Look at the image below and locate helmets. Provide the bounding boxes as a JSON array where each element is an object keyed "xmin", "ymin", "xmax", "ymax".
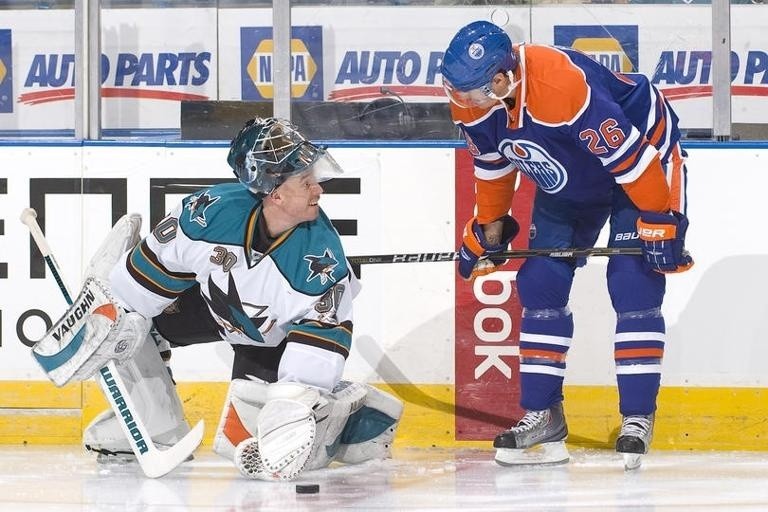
[
  {"xmin": 441, "ymin": 21, "xmax": 518, "ymax": 108},
  {"xmin": 227, "ymin": 117, "xmax": 344, "ymax": 195}
]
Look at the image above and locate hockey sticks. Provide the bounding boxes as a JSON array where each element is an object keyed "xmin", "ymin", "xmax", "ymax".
[{"xmin": 20, "ymin": 207, "xmax": 204, "ymax": 478}]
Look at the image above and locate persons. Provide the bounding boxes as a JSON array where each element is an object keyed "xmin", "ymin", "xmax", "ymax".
[
  {"xmin": 434, "ymin": 17, "xmax": 697, "ymax": 459},
  {"xmin": 79, "ymin": 114, "xmax": 401, "ymax": 483}
]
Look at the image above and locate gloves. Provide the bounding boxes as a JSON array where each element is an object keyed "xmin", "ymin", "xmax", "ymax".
[
  {"xmin": 636, "ymin": 211, "xmax": 694, "ymax": 273},
  {"xmin": 459, "ymin": 215, "xmax": 519, "ymax": 280}
]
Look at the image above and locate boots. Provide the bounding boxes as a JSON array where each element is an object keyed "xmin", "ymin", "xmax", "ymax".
[
  {"xmin": 616, "ymin": 411, "xmax": 655, "ymax": 455},
  {"xmin": 493, "ymin": 400, "xmax": 569, "ymax": 451}
]
